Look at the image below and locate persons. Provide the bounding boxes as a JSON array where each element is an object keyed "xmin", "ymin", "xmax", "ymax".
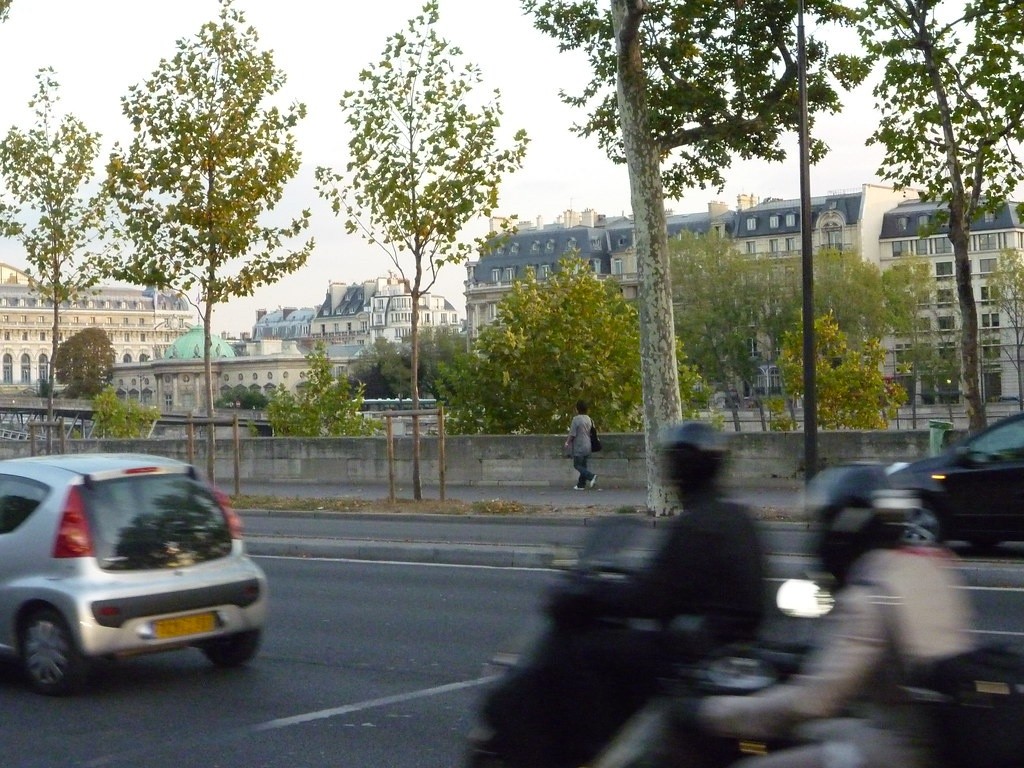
[
  {"xmin": 516, "ymin": 422, "xmax": 771, "ymax": 768},
  {"xmin": 665, "ymin": 462, "xmax": 981, "ymax": 768},
  {"xmin": 564, "ymin": 399, "xmax": 600, "ymax": 490}
]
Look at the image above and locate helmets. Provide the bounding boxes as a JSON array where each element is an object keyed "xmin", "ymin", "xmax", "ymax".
[
  {"xmin": 662, "ymin": 419, "xmax": 733, "ymax": 467},
  {"xmin": 807, "ymin": 461, "xmax": 925, "ymax": 530}
]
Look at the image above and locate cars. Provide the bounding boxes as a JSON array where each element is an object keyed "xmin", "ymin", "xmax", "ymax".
[
  {"xmin": 0, "ymin": 453, "xmax": 268, "ymax": 695},
  {"xmin": 878, "ymin": 412, "xmax": 1024, "ymax": 546}
]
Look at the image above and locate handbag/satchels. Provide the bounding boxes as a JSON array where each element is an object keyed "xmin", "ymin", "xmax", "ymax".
[{"xmin": 590, "ymin": 419, "xmax": 602, "ymax": 452}]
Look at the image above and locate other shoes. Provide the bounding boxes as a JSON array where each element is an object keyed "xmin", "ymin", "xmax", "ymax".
[
  {"xmin": 589, "ymin": 474, "xmax": 598, "ymax": 488},
  {"xmin": 573, "ymin": 485, "xmax": 585, "ymax": 491}
]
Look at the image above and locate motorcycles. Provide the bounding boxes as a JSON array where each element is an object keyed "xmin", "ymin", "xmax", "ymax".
[{"xmin": 469, "ymin": 511, "xmax": 1024, "ymax": 768}]
